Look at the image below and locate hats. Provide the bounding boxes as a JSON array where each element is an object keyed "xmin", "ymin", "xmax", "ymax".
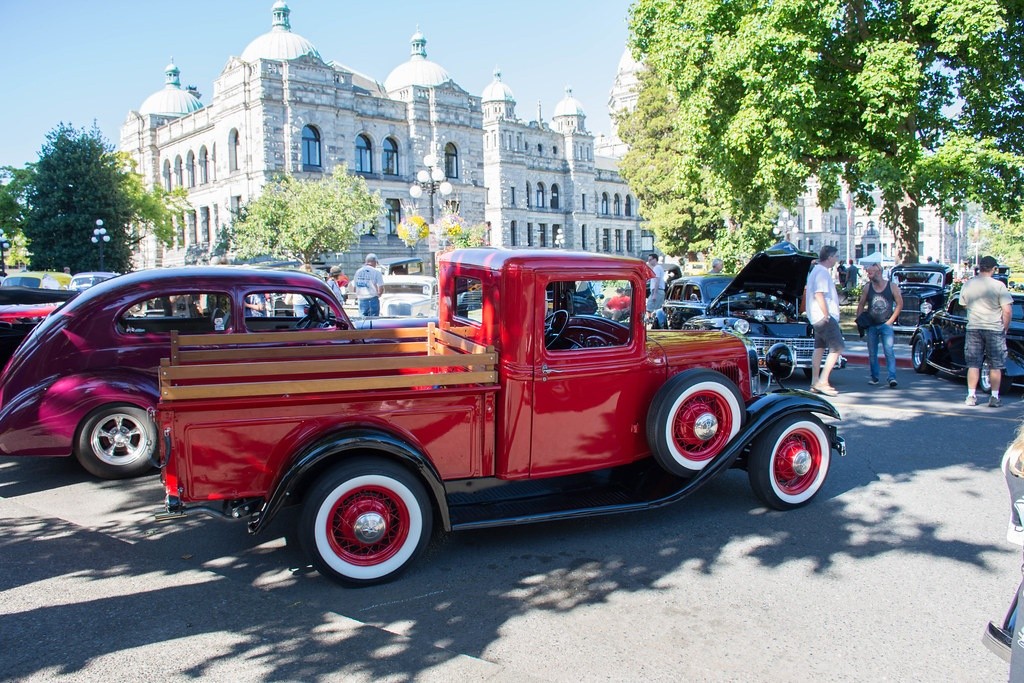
[
  {"xmin": 299, "ymin": 264, "xmax": 312, "ymax": 272},
  {"xmin": 366, "ymin": 253, "xmax": 381, "ymax": 265},
  {"xmin": 980, "ymin": 256, "xmax": 999, "ymax": 269},
  {"xmin": 329, "ymin": 266, "xmax": 340, "ymax": 277},
  {"xmin": 616, "ymin": 286, "xmax": 626, "ymax": 293}
]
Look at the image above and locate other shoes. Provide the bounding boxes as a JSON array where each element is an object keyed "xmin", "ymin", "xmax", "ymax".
[
  {"xmin": 868, "ymin": 379, "xmax": 879, "ymax": 385},
  {"xmin": 809, "ymin": 385, "xmax": 822, "ymax": 394},
  {"xmin": 889, "ymin": 379, "xmax": 897, "ymax": 388},
  {"xmin": 813, "ymin": 382, "xmax": 838, "ymax": 397}
]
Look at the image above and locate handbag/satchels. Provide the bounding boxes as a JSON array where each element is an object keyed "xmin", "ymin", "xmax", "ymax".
[{"xmin": 285, "ymin": 294, "xmax": 293, "ymax": 306}]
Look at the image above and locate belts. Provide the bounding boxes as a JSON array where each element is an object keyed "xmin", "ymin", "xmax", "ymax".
[{"xmin": 652, "ymin": 288, "xmax": 664, "ymax": 291}]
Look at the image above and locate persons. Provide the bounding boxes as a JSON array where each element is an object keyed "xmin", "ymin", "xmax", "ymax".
[
  {"xmin": 708, "ymin": 258, "xmax": 724, "ymax": 273},
  {"xmin": 200, "ymin": 256, "xmax": 229, "ymax": 312},
  {"xmin": 962, "ymin": 263, "xmax": 967, "ymax": 274},
  {"xmin": 64, "ymin": 267, "xmax": 70, "ymax": 274},
  {"xmin": 283, "ymin": 264, "xmax": 313, "ymax": 317},
  {"xmin": 353, "ymin": 253, "xmax": 384, "ymax": 317},
  {"xmin": 837, "ymin": 261, "xmax": 847, "ymax": 288},
  {"xmin": 856, "ymin": 264, "xmax": 904, "ymax": 388},
  {"xmin": 808, "ymin": 259, "xmax": 817, "ymax": 275},
  {"xmin": 927, "ymin": 256, "xmax": 940, "ymax": 264},
  {"xmin": 241, "ymin": 264, "xmax": 268, "ymax": 317},
  {"xmin": 805, "ymin": 246, "xmax": 846, "ymax": 396},
  {"xmin": 645, "ymin": 254, "xmax": 666, "ymax": 322},
  {"xmin": 317, "ymin": 266, "xmax": 344, "ymax": 317},
  {"xmin": 602, "ymin": 286, "xmax": 631, "ymax": 322},
  {"xmin": 845, "ymin": 259, "xmax": 861, "ymax": 288},
  {"xmin": 985, "ymin": 424, "xmax": 1024, "ymax": 683},
  {"xmin": 958, "ymin": 256, "xmax": 1014, "ymax": 408}
]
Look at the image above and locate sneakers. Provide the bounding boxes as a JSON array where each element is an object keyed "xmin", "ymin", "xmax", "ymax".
[
  {"xmin": 988, "ymin": 396, "xmax": 1003, "ymax": 407},
  {"xmin": 965, "ymin": 396, "xmax": 978, "ymax": 406}
]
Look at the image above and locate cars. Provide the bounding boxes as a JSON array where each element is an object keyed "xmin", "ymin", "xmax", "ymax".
[
  {"xmin": 0, "ymin": 268, "xmax": 123, "ymax": 357},
  {"xmin": 616, "ymin": 242, "xmax": 1024, "ymax": 402},
  {"xmin": 0, "ymin": 263, "xmax": 368, "ymax": 481},
  {"xmin": 347, "ymin": 254, "xmax": 440, "ymax": 321}
]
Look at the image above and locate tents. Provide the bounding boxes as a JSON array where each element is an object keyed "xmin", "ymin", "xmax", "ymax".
[{"xmin": 861, "ymin": 251, "xmax": 895, "ymax": 265}]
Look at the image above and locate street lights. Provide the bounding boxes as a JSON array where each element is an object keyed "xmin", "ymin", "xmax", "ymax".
[{"xmin": 90, "ymin": 219, "xmax": 111, "ymax": 272}]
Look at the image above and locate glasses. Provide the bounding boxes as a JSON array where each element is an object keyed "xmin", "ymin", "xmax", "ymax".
[{"xmin": 872, "ymin": 264, "xmax": 880, "ymax": 271}]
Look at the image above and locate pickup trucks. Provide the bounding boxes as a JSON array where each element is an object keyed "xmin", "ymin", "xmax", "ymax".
[{"xmin": 149, "ymin": 244, "xmax": 850, "ymax": 592}]
